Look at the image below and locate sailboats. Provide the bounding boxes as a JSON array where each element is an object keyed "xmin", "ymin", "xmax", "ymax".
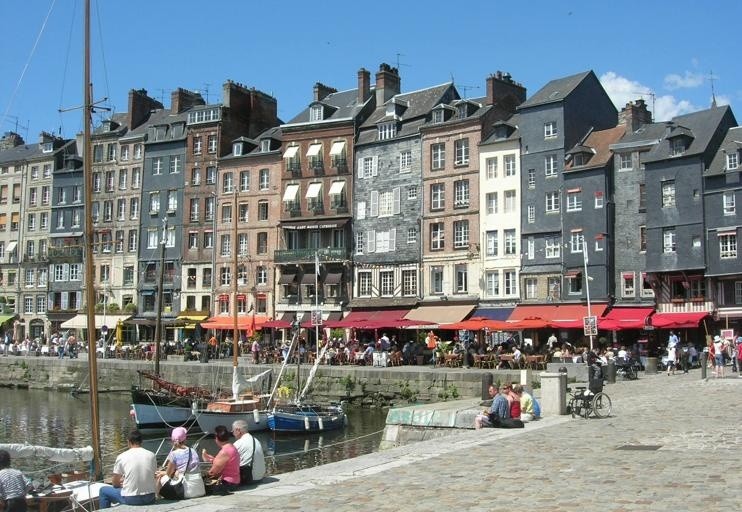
[
  {"xmin": 131, "ymin": 215, "xmax": 271, "ymax": 433},
  {"xmin": 267, "ymin": 321, "xmax": 347, "ymax": 434},
  {"xmin": 192, "ymin": 186, "xmax": 300, "ymax": 437}
]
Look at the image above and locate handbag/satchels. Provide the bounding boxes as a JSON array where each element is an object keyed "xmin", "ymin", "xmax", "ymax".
[
  {"xmin": 159, "ymin": 479, "xmax": 184, "ymax": 500},
  {"xmin": 240, "ymin": 465, "xmax": 253, "ymax": 485},
  {"xmin": 204, "ymin": 476, "xmax": 225, "ymax": 493}
]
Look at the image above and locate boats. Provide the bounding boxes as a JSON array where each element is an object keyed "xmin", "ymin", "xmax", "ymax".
[{"xmin": 135, "ymin": 425, "xmax": 348, "ymax": 473}]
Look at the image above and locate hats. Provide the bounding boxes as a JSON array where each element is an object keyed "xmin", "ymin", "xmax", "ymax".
[{"xmin": 171, "ymin": 426, "xmax": 187, "ymax": 443}]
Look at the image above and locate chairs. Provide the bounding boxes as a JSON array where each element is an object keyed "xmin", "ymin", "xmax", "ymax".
[
  {"xmin": 262, "ymin": 346, "xmax": 402, "ymax": 367},
  {"xmin": 442, "ymin": 350, "xmax": 625, "ymax": 370},
  {"xmin": 105, "ymin": 345, "xmax": 145, "ymax": 360}
]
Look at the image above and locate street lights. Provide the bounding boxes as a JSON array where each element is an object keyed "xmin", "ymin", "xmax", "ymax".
[{"xmin": 98, "ymin": 262, "xmax": 113, "ymax": 359}]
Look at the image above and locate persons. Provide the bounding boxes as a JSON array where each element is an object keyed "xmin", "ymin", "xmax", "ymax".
[
  {"xmin": 475, "ymin": 382, "xmax": 540, "ymax": 430},
  {"xmin": 230, "ymin": 419, "xmax": 266, "ymax": 485},
  {"xmin": 98, "ymin": 430, "xmax": 158, "ymax": 509},
  {"xmin": 0, "ymin": 448, "xmax": 33, "ymax": 512},
  {"xmin": 202, "ymin": 429, "xmax": 241, "ymax": 494},
  {"xmin": 155, "ymin": 426, "xmax": 206, "ymax": 499}
]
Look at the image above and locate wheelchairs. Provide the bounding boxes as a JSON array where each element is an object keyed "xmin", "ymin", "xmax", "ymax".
[
  {"xmin": 616, "ymin": 362, "xmax": 638, "ymax": 381},
  {"xmin": 570, "ymin": 384, "xmax": 612, "ymax": 420}
]
